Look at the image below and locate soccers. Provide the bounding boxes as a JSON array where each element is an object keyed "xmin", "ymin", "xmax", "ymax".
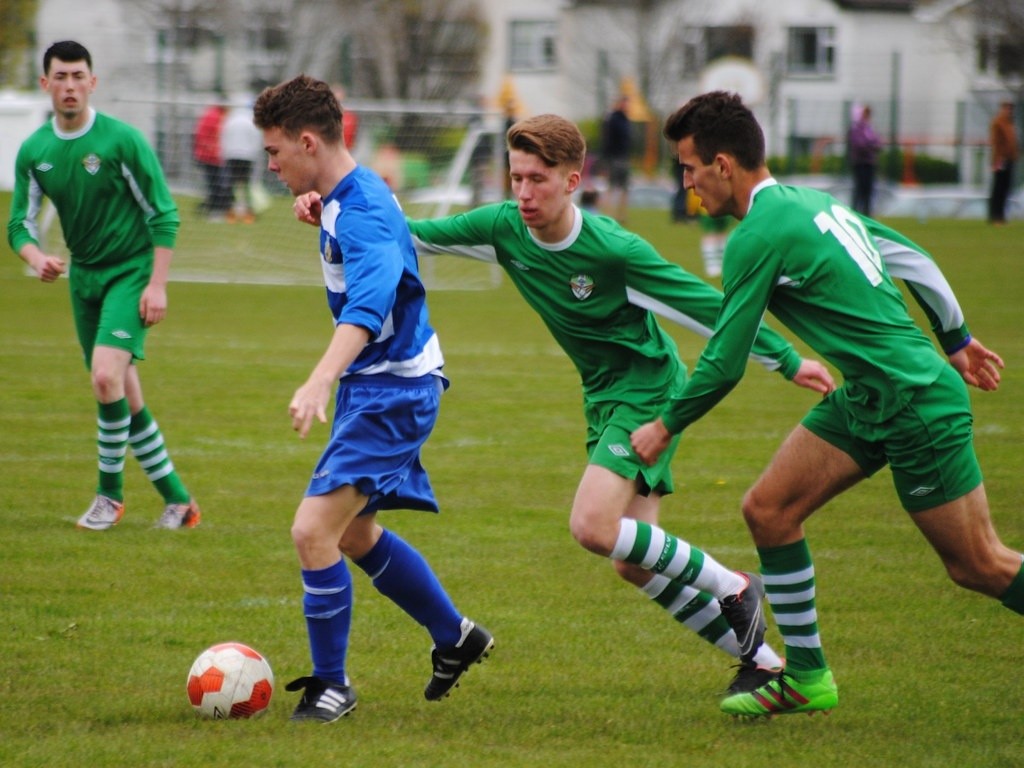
[{"xmin": 185, "ymin": 641, "xmax": 274, "ymax": 723}]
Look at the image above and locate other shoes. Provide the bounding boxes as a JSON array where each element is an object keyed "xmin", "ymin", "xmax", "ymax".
[
  {"xmin": 226, "ymin": 213, "xmax": 241, "ymax": 224},
  {"xmin": 243, "ymin": 214, "xmax": 255, "ymax": 223},
  {"xmin": 208, "ymin": 211, "xmax": 224, "ymax": 224},
  {"xmin": 193, "ymin": 204, "xmax": 207, "ymax": 218}
]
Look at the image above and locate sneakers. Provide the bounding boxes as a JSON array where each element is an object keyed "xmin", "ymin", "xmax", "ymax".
[
  {"xmin": 424, "ymin": 617, "xmax": 494, "ymax": 702},
  {"xmin": 718, "ymin": 571, "xmax": 768, "ymax": 660},
  {"xmin": 148, "ymin": 497, "xmax": 201, "ymax": 530},
  {"xmin": 75, "ymin": 497, "xmax": 126, "ymax": 531},
  {"xmin": 719, "ymin": 669, "xmax": 839, "ymax": 719},
  {"xmin": 284, "ymin": 675, "xmax": 358, "ymax": 725},
  {"xmin": 723, "ymin": 659, "xmax": 789, "ymax": 724}
]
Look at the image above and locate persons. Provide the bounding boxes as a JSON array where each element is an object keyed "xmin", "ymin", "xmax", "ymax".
[
  {"xmin": 631, "ymin": 90, "xmax": 1023, "ymax": 718},
  {"xmin": 194, "ymin": 105, "xmax": 261, "ymax": 226},
  {"xmin": 847, "ymin": 106, "xmax": 884, "ymax": 218},
  {"xmin": 582, "ymin": 96, "xmax": 633, "ymax": 228},
  {"xmin": 988, "ymin": 101, "xmax": 1019, "ymax": 221},
  {"xmin": 5, "ymin": 40, "xmax": 203, "ymax": 529},
  {"xmin": 292, "ymin": 115, "xmax": 834, "ymax": 694},
  {"xmin": 254, "ymin": 74, "xmax": 494, "ymax": 725}
]
